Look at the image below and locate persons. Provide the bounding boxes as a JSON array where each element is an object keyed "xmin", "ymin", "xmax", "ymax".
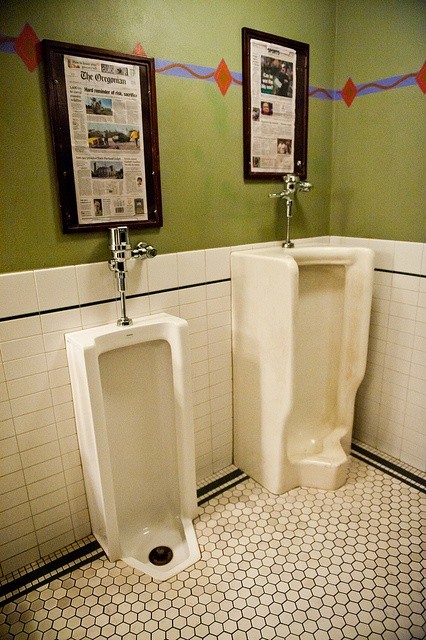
[
  {"xmin": 277, "ymin": 140, "xmax": 288, "ymax": 154},
  {"xmin": 275, "ymin": 64, "xmax": 291, "ymax": 97},
  {"xmin": 137, "ymin": 177, "xmax": 142, "ymax": 185},
  {"xmin": 263, "ymin": 102, "xmax": 271, "ymax": 114}
]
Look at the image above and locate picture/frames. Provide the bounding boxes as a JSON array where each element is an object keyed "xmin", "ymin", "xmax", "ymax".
[
  {"xmin": 241, "ymin": 26, "xmax": 310, "ymax": 182},
  {"xmin": 40, "ymin": 39, "xmax": 163, "ymax": 233}
]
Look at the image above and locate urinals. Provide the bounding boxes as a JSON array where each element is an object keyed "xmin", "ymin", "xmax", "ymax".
[
  {"xmin": 230, "ymin": 245, "xmax": 375, "ymax": 495},
  {"xmin": 65, "ymin": 312, "xmax": 205, "ymax": 583}
]
